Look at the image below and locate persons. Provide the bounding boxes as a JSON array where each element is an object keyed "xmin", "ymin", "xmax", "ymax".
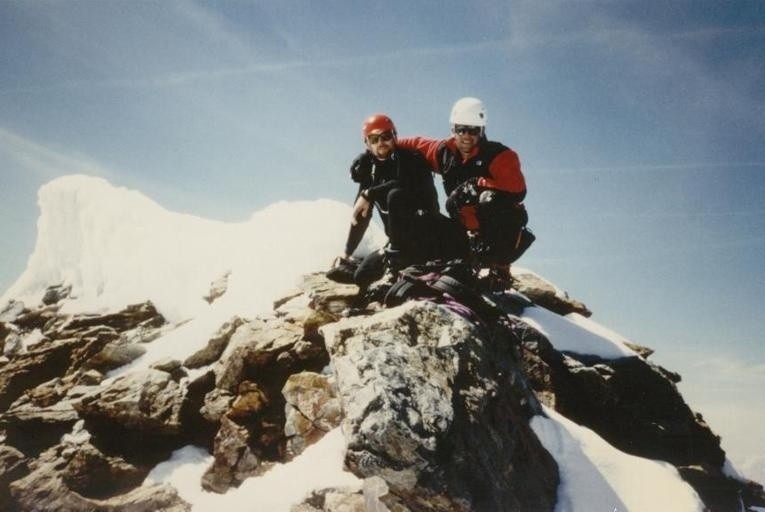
[
  {"xmin": 333, "ymin": 113, "xmax": 471, "ymax": 298},
  {"xmin": 349, "ymin": 95, "xmax": 527, "ymax": 295}
]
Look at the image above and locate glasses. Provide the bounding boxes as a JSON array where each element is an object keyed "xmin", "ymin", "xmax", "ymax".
[
  {"xmin": 364, "ymin": 131, "xmax": 394, "ymax": 143},
  {"xmin": 452, "ymin": 125, "xmax": 483, "ymax": 136}
]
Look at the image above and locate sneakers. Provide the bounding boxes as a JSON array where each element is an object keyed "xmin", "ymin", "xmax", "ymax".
[
  {"xmin": 509, "ymin": 228, "xmax": 536, "ymax": 263},
  {"xmin": 476, "ymin": 266, "xmax": 515, "ymax": 292},
  {"xmin": 366, "ymin": 265, "xmax": 400, "ymax": 293}
]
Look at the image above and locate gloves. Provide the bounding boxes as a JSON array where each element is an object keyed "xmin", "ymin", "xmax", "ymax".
[
  {"xmin": 444, "ymin": 176, "xmax": 479, "ymax": 216},
  {"xmin": 348, "ymin": 150, "xmax": 374, "ymax": 185}
]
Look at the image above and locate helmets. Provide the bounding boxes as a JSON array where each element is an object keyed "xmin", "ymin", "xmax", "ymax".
[
  {"xmin": 362, "ymin": 113, "xmax": 393, "ymax": 137},
  {"xmin": 448, "ymin": 98, "xmax": 486, "ymax": 127}
]
